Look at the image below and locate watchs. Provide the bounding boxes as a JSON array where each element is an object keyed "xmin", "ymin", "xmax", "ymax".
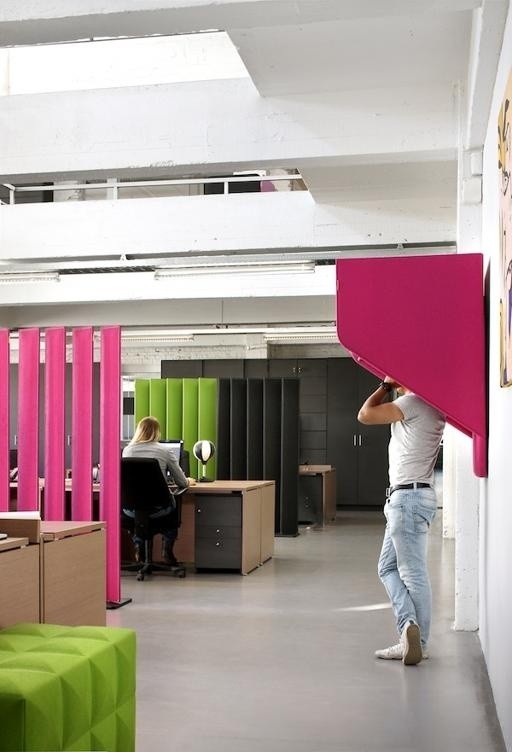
[{"xmin": 380, "ymin": 382, "xmax": 392, "ymax": 392}]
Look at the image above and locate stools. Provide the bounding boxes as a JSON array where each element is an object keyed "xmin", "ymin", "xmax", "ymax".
[{"xmin": 1, "ymin": 623, "xmax": 140, "ymax": 752}]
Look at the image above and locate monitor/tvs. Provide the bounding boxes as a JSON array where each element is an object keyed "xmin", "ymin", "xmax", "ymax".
[{"xmin": 158, "ymin": 440, "xmax": 183, "ymax": 478}]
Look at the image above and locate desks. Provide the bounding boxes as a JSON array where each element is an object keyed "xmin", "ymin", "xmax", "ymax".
[
  {"xmin": 0, "ymin": 508, "xmax": 109, "ymax": 626},
  {"xmin": 298, "ymin": 463, "xmax": 337, "ymax": 529},
  {"xmin": 7, "ymin": 478, "xmax": 276, "ymax": 576}
]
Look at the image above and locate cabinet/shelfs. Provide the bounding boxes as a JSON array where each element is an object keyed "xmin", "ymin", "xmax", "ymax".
[
  {"xmin": 298, "ymin": 473, "xmax": 322, "ymax": 525},
  {"xmin": 193, "ymin": 492, "xmax": 242, "ymax": 573}
]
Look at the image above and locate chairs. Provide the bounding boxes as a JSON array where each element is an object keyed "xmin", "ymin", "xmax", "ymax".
[{"xmin": 120, "ymin": 456, "xmax": 186, "ymax": 582}]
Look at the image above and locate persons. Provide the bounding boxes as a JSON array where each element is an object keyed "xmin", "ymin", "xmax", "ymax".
[
  {"xmin": 122, "ymin": 416, "xmax": 196, "ymax": 565},
  {"xmin": 358, "ymin": 375, "xmax": 445, "ymax": 665}
]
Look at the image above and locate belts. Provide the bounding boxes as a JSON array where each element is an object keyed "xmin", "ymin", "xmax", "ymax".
[{"xmin": 383, "ymin": 482, "xmax": 431, "ymax": 500}]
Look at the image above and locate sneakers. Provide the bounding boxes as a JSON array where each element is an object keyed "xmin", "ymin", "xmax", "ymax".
[
  {"xmin": 374, "ymin": 642, "xmax": 429, "ymax": 659},
  {"xmin": 400, "ymin": 618, "xmax": 423, "ymax": 665}
]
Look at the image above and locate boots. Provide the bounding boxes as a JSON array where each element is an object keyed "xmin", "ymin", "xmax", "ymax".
[
  {"xmin": 161, "ymin": 538, "xmax": 179, "ymax": 567},
  {"xmin": 135, "ymin": 544, "xmax": 146, "ymax": 563}
]
